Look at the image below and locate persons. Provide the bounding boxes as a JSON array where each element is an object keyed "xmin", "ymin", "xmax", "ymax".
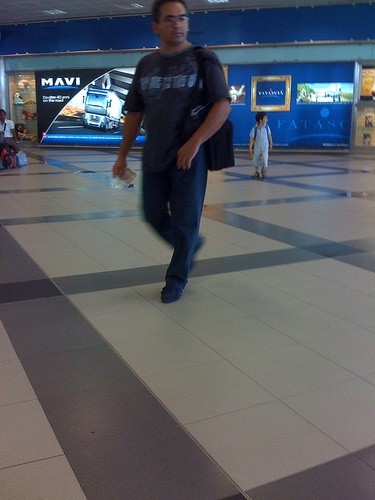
[
  {"xmin": 0, "ymin": 109, "xmax": 20, "ymax": 149},
  {"xmin": 248, "ymin": 112, "xmax": 273, "ymax": 178},
  {"xmin": 112, "ymin": 0, "xmax": 232, "ymax": 303}
]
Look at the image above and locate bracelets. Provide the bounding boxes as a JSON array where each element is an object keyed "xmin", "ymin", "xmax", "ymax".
[{"xmin": 16, "ymin": 142, "xmax": 18, "ymax": 143}]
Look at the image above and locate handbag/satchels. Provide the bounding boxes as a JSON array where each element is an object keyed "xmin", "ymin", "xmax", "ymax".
[{"xmin": 203, "ymin": 120, "xmax": 235, "ymax": 171}]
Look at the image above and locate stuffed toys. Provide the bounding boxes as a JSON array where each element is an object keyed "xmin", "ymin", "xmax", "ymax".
[
  {"xmin": 13, "ymin": 93, "xmax": 29, "ymax": 104},
  {"xmin": 22, "ymin": 111, "xmax": 36, "ymax": 121},
  {"xmin": 15, "ymin": 123, "xmax": 34, "ymax": 140}
]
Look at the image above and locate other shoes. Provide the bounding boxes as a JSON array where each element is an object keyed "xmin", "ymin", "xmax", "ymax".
[
  {"xmin": 261, "ymin": 169, "xmax": 266, "ymax": 179},
  {"xmin": 189, "ymin": 236, "xmax": 207, "ymax": 270},
  {"xmin": 251, "ymin": 172, "xmax": 260, "ymax": 178},
  {"xmin": 161, "ymin": 285, "xmax": 183, "ymax": 303}
]
